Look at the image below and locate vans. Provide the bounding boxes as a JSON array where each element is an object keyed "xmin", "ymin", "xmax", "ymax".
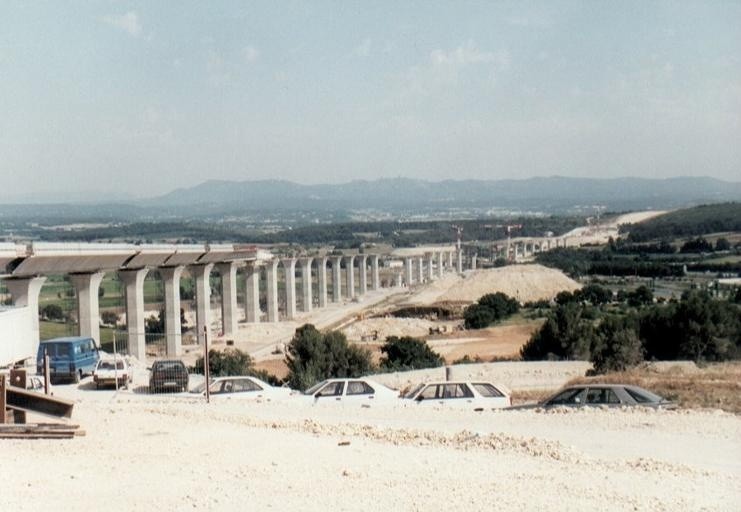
[{"xmin": 36, "ymin": 336, "xmax": 99, "ymax": 383}]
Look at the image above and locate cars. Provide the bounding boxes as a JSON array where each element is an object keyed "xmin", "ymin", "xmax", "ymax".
[
  {"xmin": 401, "ymin": 378, "xmax": 512, "ymax": 412},
  {"xmin": 501, "ymin": 383, "xmax": 677, "ymax": 412},
  {"xmin": 92, "ymin": 357, "xmax": 132, "ymax": 388},
  {"xmin": 300, "ymin": 377, "xmax": 399, "ymax": 405},
  {"xmin": 178, "ymin": 374, "xmax": 293, "ymax": 399},
  {"xmin": 147, "ymin": 360, "xmax": 189, "ymax": 393}
]
